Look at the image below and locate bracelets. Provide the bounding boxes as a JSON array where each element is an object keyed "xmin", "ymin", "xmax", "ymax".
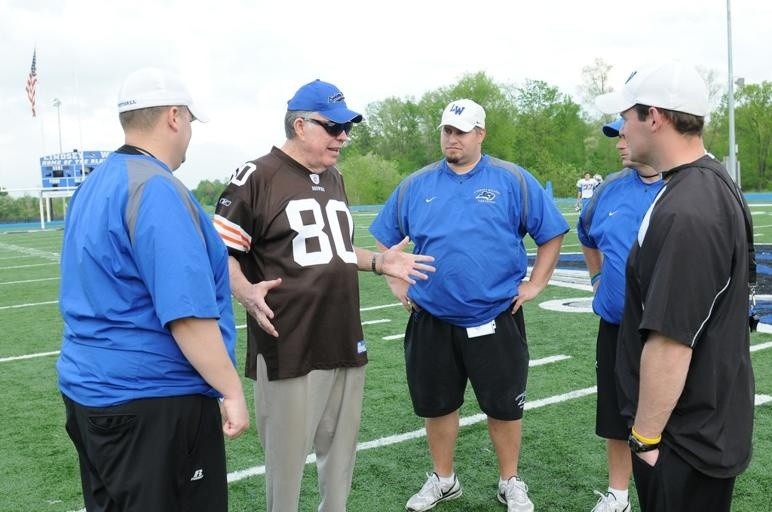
[
  {"xmin": 370, "ymin": 253, "xmax": 380, "ymax": 275},
  {"xmin": 632, "ymin": 425, "xmax": 663, "ymax": 445},
  {"xmin": 590, "ymin": 273, "xmax": 603, "ymax": 285}
]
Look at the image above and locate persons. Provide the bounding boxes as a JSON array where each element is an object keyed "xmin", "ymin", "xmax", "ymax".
[
  {"xmin": 575, "ymin": 172, "xmax": 603, "ymax": 211},
  {"xmin": 57, "ymin": 67, "xmax": 250, "ymax": 512},
  {"xmin": 595, "ymin": 59, "xmax": 756, "ymax": 511},
  {"xmin": 212, "ymin": 79, "xmax": 436, "ymax": 511},
  {"xmin": 578, "ymin": 115, "xmax": 666, "ymax": 512},
  {"xmin": 367, "ymin": 98, "xmax": 570, "ymax": 512}
]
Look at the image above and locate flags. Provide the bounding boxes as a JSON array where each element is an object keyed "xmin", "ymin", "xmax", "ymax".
[{"xmin": 25, "ymin": 46, "xmax": 37, "ymax": 119}]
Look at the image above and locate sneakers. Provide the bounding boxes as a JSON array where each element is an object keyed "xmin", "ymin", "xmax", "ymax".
[
  {"xmin": 590, "ymin": 488, "xmax": 631, "ymax": 512},
  {"xmin": 497, "ymin": 476, "xmax": 534, "ymax": 512},
  {"xmin": 405, "ymin": 472, "xmax": 462, "ymax": 511}
]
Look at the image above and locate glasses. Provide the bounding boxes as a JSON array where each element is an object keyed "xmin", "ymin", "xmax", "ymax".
[{"xmin": 310, "ymin": 118, "xmax": 353, "ymax": 134}]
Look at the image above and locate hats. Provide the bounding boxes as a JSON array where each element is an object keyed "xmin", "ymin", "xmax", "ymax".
[
  {"xmin": 596, "ymin": 60, "xmax": 708, "ymax": 116},
  {"xmin": 437, "ymin": 99, "xmax": 486, "ymax": 133},
  {"xmin": 287, "ymin": 78, "xmax": 363, "ymax": 123},
  {"xmin": 119, "ymin": 69, "xmax": 208, "ymax": 123},
  {"xmin": 602, "ymin": 119, "xmax": 623, "ymax": 137}
]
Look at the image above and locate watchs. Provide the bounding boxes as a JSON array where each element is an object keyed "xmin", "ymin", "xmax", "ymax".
[{"xmin": 627, "ymin": 434, "xmax": 660, "ymax": 453}]
